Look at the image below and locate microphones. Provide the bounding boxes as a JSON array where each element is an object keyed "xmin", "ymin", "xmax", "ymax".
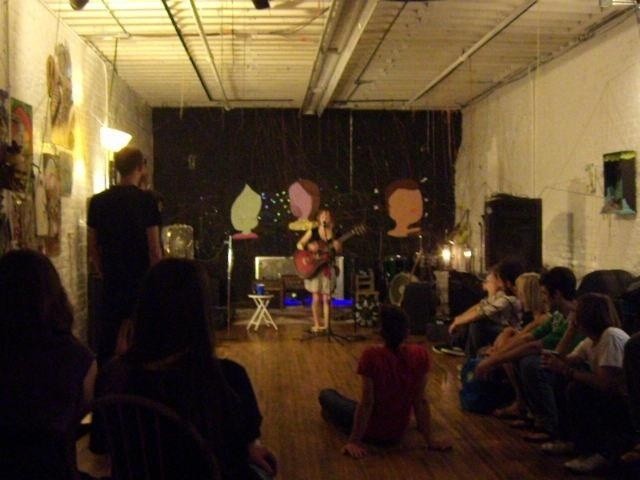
[{"xmin": 322, "ymin": 218, "xmax": 327, "ymax": 229}]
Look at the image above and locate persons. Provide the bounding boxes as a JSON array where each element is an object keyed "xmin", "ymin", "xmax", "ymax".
[
  {"xmin": 320, "ymin": 304, "xmax": 452, "ymax": 459},
  {"xmin": 87, "ymin": 258, "xmax": 279, "ymax": 480},
  {"xmin": 0, "ymin": 250, "xmax": 98, "ymax": 480},
  {"xmin": 87, "ymin": 148, "xmax": 163, "ymax": 455},
  {"xmin": 297, "ymin": 209, "xmax": 343, "ymax": 333}
]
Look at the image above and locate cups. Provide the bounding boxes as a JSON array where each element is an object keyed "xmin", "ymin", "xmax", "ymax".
[{"xmin": 256, "ymin": 283, "xmax": 265, "ymax": 296}]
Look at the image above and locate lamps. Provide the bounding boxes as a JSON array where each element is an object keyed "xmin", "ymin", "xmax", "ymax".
[
  {"xmin": 97, "ymin": 122, "xmax": 132, "ymax": 154},
  {"xmin": 441, "ymin": 207, "xmax": 473, "ymax": 261}
]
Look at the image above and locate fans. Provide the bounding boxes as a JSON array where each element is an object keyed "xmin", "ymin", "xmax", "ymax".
[{"xmin": 388, "ymin": 272, "xmax": 419, "ymax": 307}]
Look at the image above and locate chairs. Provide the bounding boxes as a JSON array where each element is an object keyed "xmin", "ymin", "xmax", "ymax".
[{"xmin": 350, "ymin": 266, "xmax": 381, "ymax": 334}]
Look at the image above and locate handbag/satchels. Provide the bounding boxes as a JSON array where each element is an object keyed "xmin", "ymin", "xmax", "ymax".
[{"xmin": 458, "ymin": 354, "xmax": 516, "ymax": 414}]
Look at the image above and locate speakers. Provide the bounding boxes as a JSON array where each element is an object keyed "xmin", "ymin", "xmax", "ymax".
[{"xmin": 484, "ymin": 198, "xmax": 542, "ymax": 272}]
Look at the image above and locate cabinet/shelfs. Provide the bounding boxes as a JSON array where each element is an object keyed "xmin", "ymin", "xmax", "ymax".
[{"xmin": 482, "ymin": 194, "xmax": 544, "ymax": 276}]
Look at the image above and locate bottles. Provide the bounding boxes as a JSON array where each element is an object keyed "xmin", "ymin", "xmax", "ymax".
[{"xmin": 568, "ymin": 337, "xmax": 594, "ymax": 359}]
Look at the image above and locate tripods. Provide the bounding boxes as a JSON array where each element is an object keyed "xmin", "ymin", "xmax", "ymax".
[{"xmin": 298, "ymin": 282, "xmax": 355, "ymax": 346}]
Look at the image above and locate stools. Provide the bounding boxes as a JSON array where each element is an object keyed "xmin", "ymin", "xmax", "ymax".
[{"xmin": 245, "ymin": 293, "xmax": 278, "ymax": 335}]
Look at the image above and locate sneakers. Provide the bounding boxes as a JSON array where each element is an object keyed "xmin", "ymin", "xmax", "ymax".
[
  {"xmin": 492, "ymin": 401, "xmax": 525, "ymax": 417},
  {"xmin": 432, "ymin": 338, "xmax": 467, "ymax": 357},
  {"xmin": 539, "ymin": 440, "xmax": 575, "ymax": 450},
  {"xmin": 563, "ymin": 455, "xmax": 607, "ymax": 472},
  {"xmin": 509, "ymin": 419, "xmax": 533, "ymax": 427},
  {"xmin": 519, "ymin": 431, "xmax": 548, "ymax": 440}
]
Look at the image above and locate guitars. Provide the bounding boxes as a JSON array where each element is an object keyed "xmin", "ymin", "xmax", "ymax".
[{"xmin": 296, "ymin": 222, "xmax": 366, "ymax": 279}]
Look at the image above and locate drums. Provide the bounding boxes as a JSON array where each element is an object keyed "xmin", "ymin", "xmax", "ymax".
[
  {"xmin": 387, "ymin": 272, "xmax": 422, "ymax": 305},
  {"xmin": 383, "ymin": 255, "xmax": 409, "ymax": 275}
]
